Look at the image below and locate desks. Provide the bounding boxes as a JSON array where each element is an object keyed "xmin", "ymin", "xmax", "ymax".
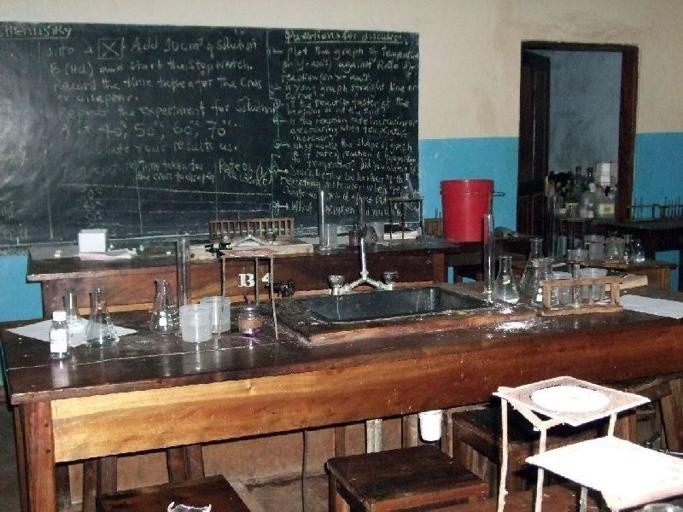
[
  {"xmin": 0, "ymin": 278, "xmax": 683, "ymax": 510},
  {"xmin": 27, "ymin": 226, "xmax": 551, "ymax": 496}
]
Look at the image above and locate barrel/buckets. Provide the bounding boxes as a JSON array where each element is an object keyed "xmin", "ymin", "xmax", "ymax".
[{"xmin": 438, "ymin": 178, "xmax": 495, "ymax": 246}]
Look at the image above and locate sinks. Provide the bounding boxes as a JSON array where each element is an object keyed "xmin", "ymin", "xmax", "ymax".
[{"xmin": 282, "ymin": 281, "xmax": 491, "ymax": 329}]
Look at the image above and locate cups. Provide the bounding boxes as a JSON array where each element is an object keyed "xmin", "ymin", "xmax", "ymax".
[
  {"xmin": 553, "ymin": 268, "xmax": 572, "ymax": 305},
  {"xmin": 320, "ymin": 224, "xmax": 337, "ymax": 247},
  {"xmin": 567, "ymin": 235, "xmax": 605, "ymax": 272},
  {"xmin": 581, "ymin": 268, "xmax": 608, "ymax": 302},
  {"xmin": 180, "ymin": 296, "xmax": 231, "ymax": 343}
]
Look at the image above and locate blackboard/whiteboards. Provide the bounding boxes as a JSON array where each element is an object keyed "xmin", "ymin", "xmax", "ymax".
[{"xmin": 0, "ymin": 21, "xmax": 419, "ymax": 256}]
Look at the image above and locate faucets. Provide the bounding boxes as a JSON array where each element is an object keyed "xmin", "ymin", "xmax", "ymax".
[{"xmin": 328, "ymin": 194, "xmax": 400, "ymax": 294}]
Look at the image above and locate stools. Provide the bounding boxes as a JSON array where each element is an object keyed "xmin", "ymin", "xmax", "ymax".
[
  {"xmin": 97, "ymin": 474, "xmax": 248, "ymax": 510},
  {"xmin": 614, "ymin": 378, "xmax": 683, "ymax": 451},
  {"xmin": 318, "ymin": 443, "xmax": 489, "ymax": 507},
  {"xmin": 446, "ymin": 406, "xmax": 597, "ymax": 494},
  {"xmin": 490, "ymin": 374, "xmax": 651, "ymax": 509},
  {"xmin": 525, "ymin": 435, "xmax": 682, "ymax": 508}
]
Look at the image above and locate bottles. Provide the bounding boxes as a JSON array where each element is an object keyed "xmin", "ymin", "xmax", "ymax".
[
  {"xmin": 149, "ymin": 279, "xmax": 179, "ymax": 334},
  {"xmin": 548, "ymin": 166, "xmax": 615, "ymax": 218},
  {"xmin": 238, "ymin": 305, "xmax": 263, "ymax": 337},
  {"xmin": 48, "ymin": 287, "xmax": 118, "ymax": 361},
  {"xmin": 349, "ymin": 223, "xmax": 363, "ymax": 249},
  {"xmin": 400, "ymin": 173, "xmax": 413, "ymax": 200},
  {"xmin": 480, "ymin": 214, "xmax": 560, "ymax": 307},
  {"xmin": 606, "ymin": 231, "xmax": 645, "ymax": 264}
]
